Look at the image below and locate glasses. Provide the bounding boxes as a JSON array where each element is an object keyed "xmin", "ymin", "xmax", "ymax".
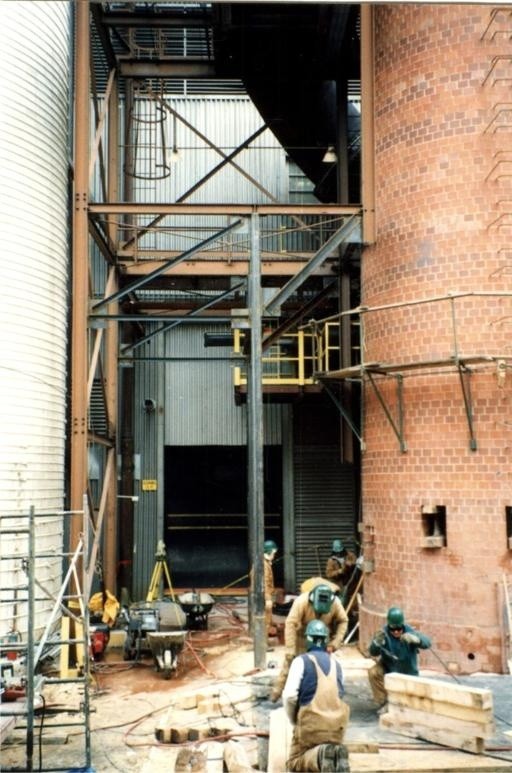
[{"xmin": 388, "ymin": 626, "xmax": 402, "ymax": 631}]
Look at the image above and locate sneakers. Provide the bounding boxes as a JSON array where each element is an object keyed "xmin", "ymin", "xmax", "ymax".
[{"xmin": 377, "ymin": 702, "xmax": 387, "ymax": 714}]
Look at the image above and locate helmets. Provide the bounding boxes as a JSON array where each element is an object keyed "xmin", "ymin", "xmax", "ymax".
[
  {"xmin": 263, "ymin": 540, "xmax": 276, "ymax": 553},
  {"xmin": 314, "ymin": 585, "xmax": 331, "ymax": 613},
  {"xmin": 332, "ymin": 540, "xmax": 344, "ymax": 552},
  {"xmin": 387, "ymin": 608, "xmax": 404, "ymax": 628},
  {"xmin": 304, "ymin": 620, "xmax": 328, "ymax": 652}
]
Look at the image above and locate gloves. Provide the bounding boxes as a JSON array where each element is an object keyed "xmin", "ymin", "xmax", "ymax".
[
  {"xmin": 400, "ymin": 633, "xmax": 420, "ymax": 645},
  {"xmin": 376, "ymin": 630, "xmax": 385, "ymax": 644},
  {"xmin": 265, "ymin": 600, "xmax": 272, "ymax": 610}
]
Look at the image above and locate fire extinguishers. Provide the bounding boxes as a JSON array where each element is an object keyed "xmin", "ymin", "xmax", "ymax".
[
  {"xmin": 94, "ymin": 629, "xmax": 106, "ymax": 661},
  {"xmin": 8, "ymin": 629, "xmax": 23, "ymax": 661}
]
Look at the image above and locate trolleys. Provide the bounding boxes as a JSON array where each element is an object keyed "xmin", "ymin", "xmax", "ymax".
[
  {"xmin": 144, "ymin": 629, "xmax": 187, "ymax": 678},
  {"xmin": 180, "ymin": 592, "xmax": 214, "ymax": 630}
]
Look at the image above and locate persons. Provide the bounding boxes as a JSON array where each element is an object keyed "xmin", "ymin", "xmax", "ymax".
[
  {"xmin": 281, "ymin": 617, "xmax": 350, "ymax": 773},
  {"xmin": 248, "ymin": 540, "xmax": 277, "ymax": 652},
  {"xmin": 367, "ymin": 607, "xmax": 431, "ymax": 716},
  {"xmin": 324, "ymin": 538, "xmax": 362, "ymax": 642},
  {"xmin": 272, "ymin": 583, "xmax": 349, "ymax": 703}
]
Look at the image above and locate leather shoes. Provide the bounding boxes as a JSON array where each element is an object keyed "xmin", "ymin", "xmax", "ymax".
[{"xmin": 319, "ymin": 744, "xmax": 350, "ymax": 773}]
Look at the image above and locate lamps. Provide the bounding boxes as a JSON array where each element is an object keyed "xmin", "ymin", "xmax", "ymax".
[
  {"xmin": 322, "ymin": 147, "xmax": 336, "ymax": 163},
  {"xmin": 168, "ymin": 114, "xmax": 182, "ymax": 162}
]
